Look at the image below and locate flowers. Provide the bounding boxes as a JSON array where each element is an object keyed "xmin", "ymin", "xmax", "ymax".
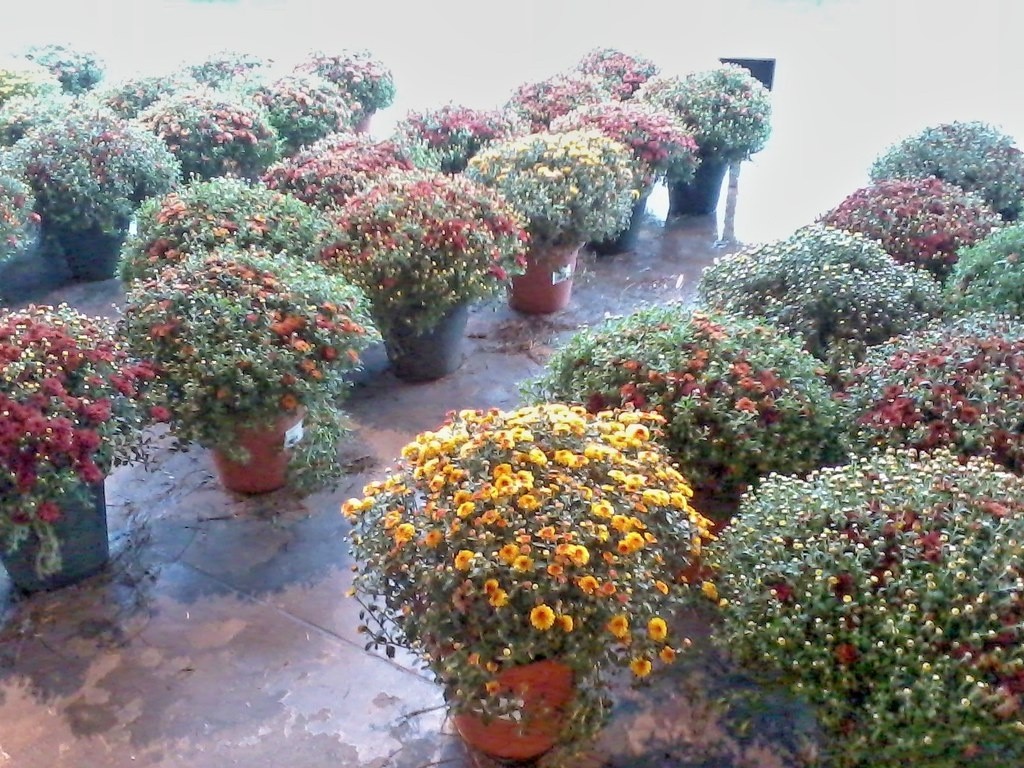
[{"xmin": 0, "ymin": 42, "xmax": 1024, "ymax": 768}]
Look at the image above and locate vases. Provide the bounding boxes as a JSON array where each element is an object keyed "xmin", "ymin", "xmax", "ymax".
[
  {"xmin": 508, "ymin": 238, "xmax": 584, "ymax": 314},
  {"xmin": 5, "ymin": 482, "xmax": 109, "ymax": 590},
  {"xmin": 386, "ymin": 305, "xmax": 474, "ymax": 380},
  {"xmin": 596, "ymin": 183, "xmax": 654, "ymax": 253},
  {"xmin": 450, "ymin": 660, "xmax": 574, "ymax": 758},
  {"xmin": 212, "ymin": 408, "xmax": 307, "ymax": 493},
  {"xmin": 668, "ymin": 164, "xmax": 732, "ymax": 217},
  {"xmin": 61, "ymin": 235, "xmax": 126, "ymax": 280},
  {"xmin": 677, "ymin": 502, "xmax": 735, "ymax": 584}
]
